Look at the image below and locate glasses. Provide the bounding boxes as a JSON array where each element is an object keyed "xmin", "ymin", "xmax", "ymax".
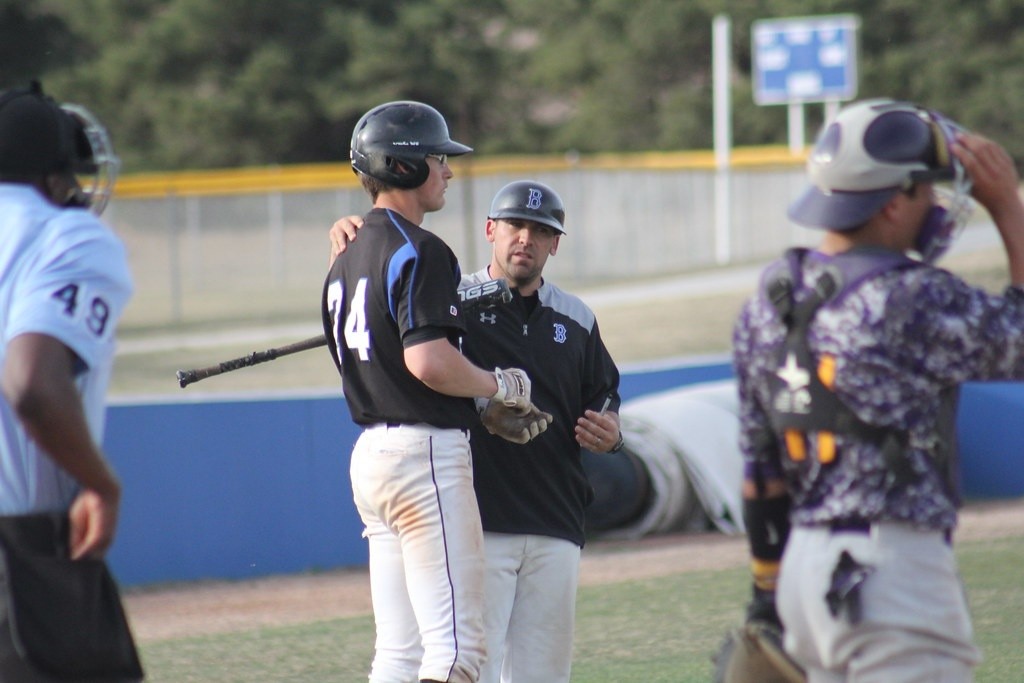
[{"xmin": 427, "ymin": 154, "xmax": 447, "ymax": 164}]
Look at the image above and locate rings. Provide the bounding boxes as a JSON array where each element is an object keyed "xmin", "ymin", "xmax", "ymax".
[{"xmin": 596, "ymin": 438, "xmax": 600, "ymax": 445}]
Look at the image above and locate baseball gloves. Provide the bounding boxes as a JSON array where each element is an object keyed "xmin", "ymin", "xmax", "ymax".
[{"xmin": 710, "ymin": 627, "xmax": 810, "ymax": 683}]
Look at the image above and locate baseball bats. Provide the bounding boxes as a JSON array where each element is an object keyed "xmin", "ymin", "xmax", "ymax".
[{"xmin": 174, "ymin": 277, "xmax": 513, "ymax": 389}]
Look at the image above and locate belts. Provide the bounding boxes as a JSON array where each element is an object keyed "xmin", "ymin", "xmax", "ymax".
[{"xmin": 0, "ymin": 513, "xmax": 69, "ymax": 545}]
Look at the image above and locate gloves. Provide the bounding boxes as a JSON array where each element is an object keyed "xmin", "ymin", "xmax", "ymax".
[
  {"xmin": 745, "ymin": 584, "xmax": 784, "ymax": 650},
  {"xmin": 488, "ymin": 367, "xmax": 531, "ymax": 409},
  {"xmin": 476, "ymin": 398, "xmax": 553, "ymax": 444}
]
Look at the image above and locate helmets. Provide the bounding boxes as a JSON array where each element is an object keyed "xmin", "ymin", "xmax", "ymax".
[
  {"xmin": 349, "ymin": 101, "xmax": 473, "ymax": 190},
  {"xmin": 0, "ymin": 79, "xmax": 121, "ymax": 217},
  {"xmin": 487, "ymin": 181, "xmax": 566, "ymax": 236},
  {"xmin": 786, "ymin": 97, "xmax": 978, "ymax": 265}
]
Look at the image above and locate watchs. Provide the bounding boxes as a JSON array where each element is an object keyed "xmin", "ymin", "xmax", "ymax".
[{"xmin": 607, "ymin": 431, "xmax": 625, "ymax": 455}]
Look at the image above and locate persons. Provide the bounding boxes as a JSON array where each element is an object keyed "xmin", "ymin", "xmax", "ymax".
[
  {"xmin": 0, "ymin": 67, "xmax": 149, "ymax": 683},
  {"xmin": 329, "ymin": 180, "xmax": 622, "ymax": 683},
  {"xmin": 320, "ymin": 100, "xmax": 554, "ymax": 683},
  {"xmin": 733, "ymin": 94, "xmax": 1024, "ymax": 683}
]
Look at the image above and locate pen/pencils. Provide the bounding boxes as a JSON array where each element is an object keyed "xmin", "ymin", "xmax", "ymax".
[{"xmin": 600, "ymin": 394, "xmax": 613, "ymax": 415}]
